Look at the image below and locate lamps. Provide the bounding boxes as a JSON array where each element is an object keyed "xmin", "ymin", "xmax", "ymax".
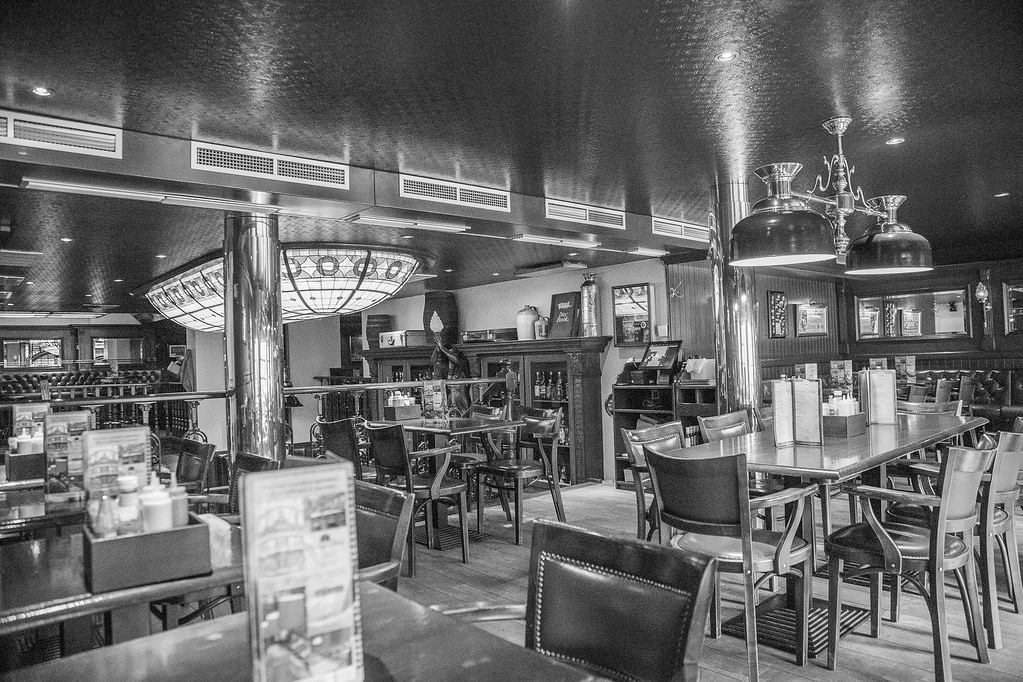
[
  {"xmin": 974, "ymin": 281, "xmax": 991, "ymax": 330},
  {"xmin": 128, "ymin": 244, "xmax": 439, "ymax": 334},
  {"xmin": 728, "ymin": 117, "xmax": 934, "ymax": 274},
  {"xmin": 284, "ymin": 396, "xmax": 304, "ymax": 408}
]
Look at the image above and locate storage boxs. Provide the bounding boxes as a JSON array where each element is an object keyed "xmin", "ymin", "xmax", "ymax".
[
  {"xmin": 5, "ymin": 451, "xmax": 46, "ymax": 481},
  {"xmin": 379, "ymin": 330, "xmax": 434, "ymax": 348},
  {"xmin": 83, "ymin": 512, "xmax": 213, "ymax": 594},
  {"xmin": 383, "ymin": 404, "xmax": 421, "ymax": 420},
  {"xmin": 687, "ymin": 359, "xmax": 715, "ymax": 380}
]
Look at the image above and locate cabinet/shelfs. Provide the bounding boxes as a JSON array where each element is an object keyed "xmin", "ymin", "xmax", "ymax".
[
  {"xmin": 675, "ymin": 381, "xmax": 717, "ymax": 438},
  {"xmin": 612, "ymin": 381, "xmax": 677, "ymax": 494},
  {"xmin": 356, "ymin": 346, "xmax": 436, "ymax": 422},
  {"xmin": 451, "ymin": 335, "xmax": 613, "ymax": 493}
]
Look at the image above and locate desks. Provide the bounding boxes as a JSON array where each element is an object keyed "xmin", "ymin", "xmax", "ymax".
[
  {"xmin": 659, "ymin": 411, "xmax": 989, "ymax": 660},
  {"xmin": 0, "ymin": 513, "xmax": 244, "ymax": 648},
  {"xmin": 0, "ymin": 577, "xmax": 613, "ymax": 682},
  {"xmin": 355, "ymin": 417, "xmax": 527, "ymax": 550},
  {"xmin": 0, "ymin": 499, "xmax": 107, "ymax": 666}
]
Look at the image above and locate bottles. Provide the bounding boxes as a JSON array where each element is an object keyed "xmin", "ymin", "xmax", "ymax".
[
  {"xmin": 382, "ymin": 370, "xmax": 435, "ymax": 407},
  {"xmin": 825, "ymin": 392, "xmax": 859, "ymax": 417},
  {"xmin": 534, "ymin": 316, "xmax": 549, "ymax": 340},
  {"xmin": 16, "ymin": 428, "xmax": 44, "ymax": 454},
  {"xmin": 92, "ymin": 470, "xmax": 189, "ymax": 541},
  {"xmin": 579, "ymin": 272, "xmax": 602, "ymax": 338},
  {"xmin": 533, "ymin": 370, "xmax": 570, "ymax": 401},
  {"xmin": 684, "ymin": 426, "xmax": 705, "ymax": 447},
  {"xmin": 516, "ymin": 306, "xmax": 538, "ymax": 341}
]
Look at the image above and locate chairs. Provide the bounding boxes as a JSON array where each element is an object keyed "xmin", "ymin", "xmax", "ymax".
[{"xmin": 0, "ymin": 376, "xmax": 1023, "ymax": 682}]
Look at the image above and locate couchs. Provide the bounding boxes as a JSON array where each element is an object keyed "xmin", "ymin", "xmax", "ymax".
[{"xmin": 818, "ymin": 370, "xmax": 1023, "ymax": 447}]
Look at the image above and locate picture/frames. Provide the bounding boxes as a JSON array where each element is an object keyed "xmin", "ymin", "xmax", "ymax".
[{"xmin": 767, "ymin": 290, "xmax": 787, "ymax": 338}]
